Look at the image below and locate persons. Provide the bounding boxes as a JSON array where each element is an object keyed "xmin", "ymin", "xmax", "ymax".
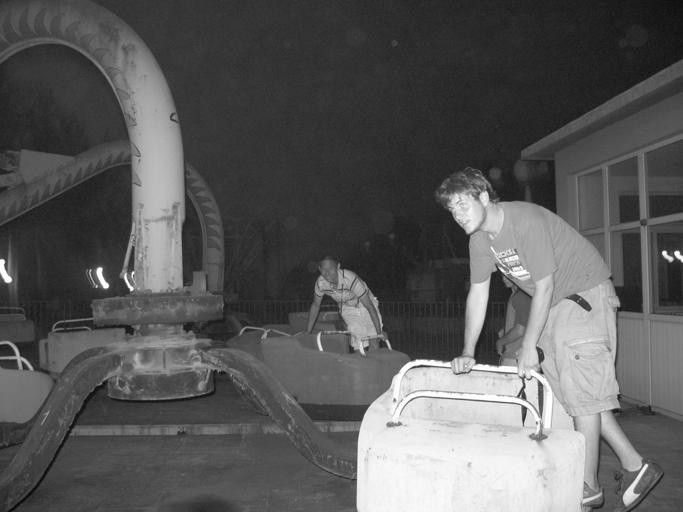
[
  {"xmin": 302, "ymin": 253, "xmax": 383, "ymax": 352},
  {"xmin": 493, "ymin": 271, "xmax": 531, "ymax": 369},
  {"xmin": 433, "ymin": 166, "xmax": 665, "ymax": 512}
]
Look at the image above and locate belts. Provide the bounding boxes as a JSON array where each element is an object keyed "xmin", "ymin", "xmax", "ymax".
[{"xmin": 564, "ymin": 293, "xmax": 595, "ymax": 311}]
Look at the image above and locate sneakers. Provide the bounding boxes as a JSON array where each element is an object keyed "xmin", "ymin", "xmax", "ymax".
[
  {"xmin": 612, "ymin": 455, "xmax": 664, "ymax": 512},
  {"xmin": 581, "ymin": 480, "xmax": 604, "ymax": 508}
]
